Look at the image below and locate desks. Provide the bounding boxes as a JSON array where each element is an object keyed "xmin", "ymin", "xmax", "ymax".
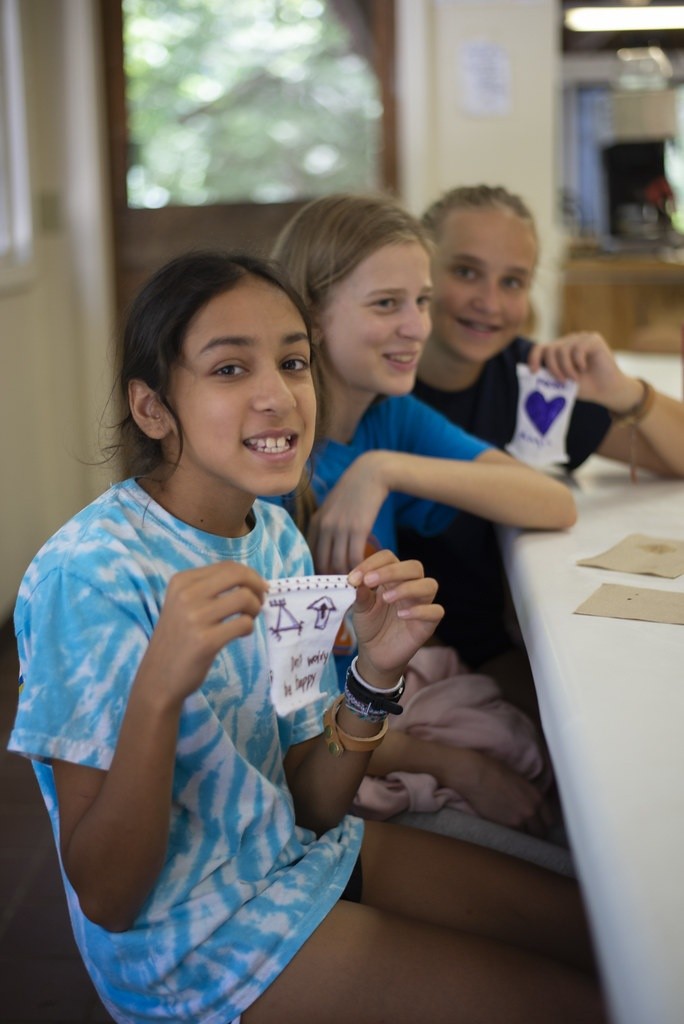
[{"xmin": 493, "ymin": 351, "xmax": 684, "ymax": 1024}]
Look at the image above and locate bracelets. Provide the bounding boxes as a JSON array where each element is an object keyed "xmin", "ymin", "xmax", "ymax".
[
  {"xmin": 322, "ymin": 693, "xmax": 389, "ymax": 760},
  {"xmin": 608, "ymin": 378, "xmax": 655, "ymax": 428},
  {"xmin": 343, "ymin": 655, "xmax": 405, "ymax": 722}
]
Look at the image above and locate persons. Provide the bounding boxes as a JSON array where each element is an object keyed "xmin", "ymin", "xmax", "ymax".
[
  {"xmin": 6, "ymin": 250, "xmax": 609, "ymax": 1024},
  {"xmin": 396, "ymin": 186, "xmax": 684, "ymax": 712},
  {"xmin": 269, "ymin": 194, "xmax": 577, "ymax": 879}
]
